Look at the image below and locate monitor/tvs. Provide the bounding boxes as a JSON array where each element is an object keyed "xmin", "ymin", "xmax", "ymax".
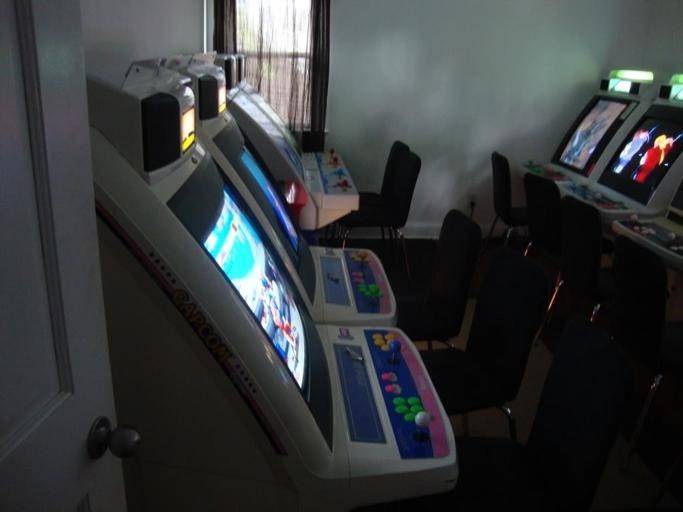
[
  {"xmin": 249, "ymin": 90, "xmax": 301, "ymax": 154},
  {"xmin": 602, "ymin": 112, "xmax": 681, "ymax": 195},
  {"xmin": 560, "ymin": 99, "xmax": 628, "ymax": 177},
  {"xmin": 203, "ymin": 189, "xmax": 311, "ymax": 403},
  {"xmin": 238, "ymin": 143, "xmax": 301, "ymax": 257},
  {"xmin": 235, "ymin": 94, "xmax": 303, "ymax": 173}
]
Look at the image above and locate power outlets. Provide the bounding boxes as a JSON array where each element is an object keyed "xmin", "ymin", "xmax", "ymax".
[{"xmin": 464, "ymin": 191, "xmax": 479, "ymax": 209}]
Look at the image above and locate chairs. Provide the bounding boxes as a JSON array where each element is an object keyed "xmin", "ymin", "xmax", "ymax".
[
  {"xmin": 421, "ymin": 258, "xmax": 547, "ymax": 443},
  {"xmin": 401, "ymin": 209, "xmax": 481, "ymax": 350},
  {"xmin": 610, "ymin": 235, "xmax": 681, "ymax": 433},
  {"xmin": 450, "ymin": 318, "xmax": 632, "ymax": 511},
  {"xmin": 336, "ymin": 140, "xmax": 421, "ymax": 279},
  {"xmin": 487, "ymin": 151, "xmax": 527, "ymax": 241},
  {"xmin": 523, "ymin": 172, "xmax": 601, "ymax": 275}
]
